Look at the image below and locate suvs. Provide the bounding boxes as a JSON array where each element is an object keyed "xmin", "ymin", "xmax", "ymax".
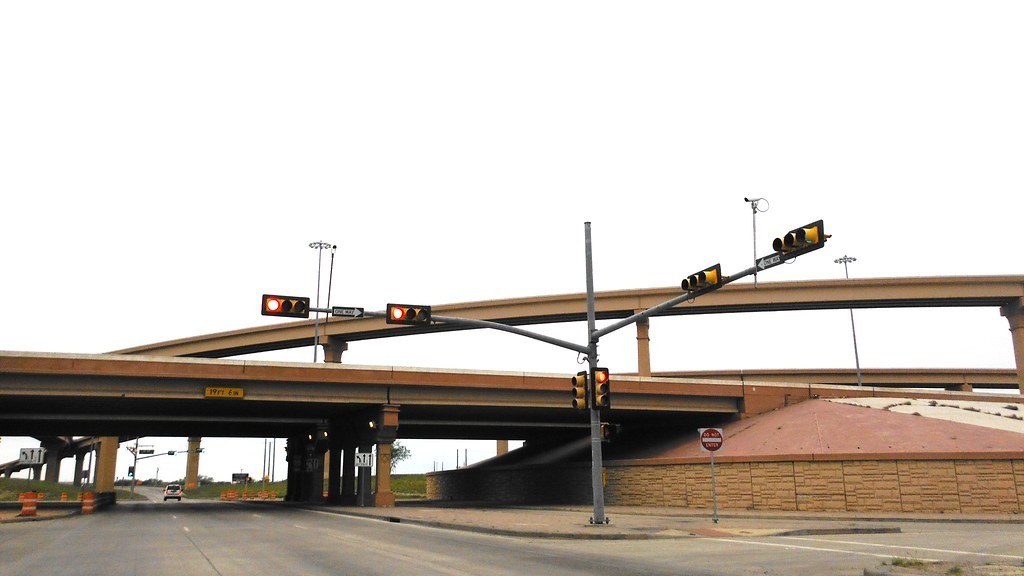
[{"xmin": 163, "ymin": 483, "xmax": 183, "ymax": 501}]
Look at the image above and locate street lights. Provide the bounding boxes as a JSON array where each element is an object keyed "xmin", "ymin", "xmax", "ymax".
[
  {"xmin": 307, "ymin": 240, "xmax": 332, "ymax": 362},
  {"xmin": 835, "ymin": 255, "xmax": 865, "ymax": 387}
]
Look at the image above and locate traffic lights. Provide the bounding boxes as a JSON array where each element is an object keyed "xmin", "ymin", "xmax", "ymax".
[
  {"xmin": 681, "ymin": 264, "xmax": 721, "ymax": 301},
  {"xmin": 385, "ymin": 302, "xmax": 432, "ymax": 327},
  {"xmin": 772, "ymin": 220, "xmax": 826, "ymax": 261},
  {"xmin": 590, "ymin": 366, "xmax": 612, "ymax": 411},
  {"xmin": 261, "ymin": 293, "xmax": 311, "ymax": 319},
  {"xmin": 128, "ymin": 466, "xmax": 134, "ymax": 477},
  {"xmin": 244, "ymin": 477, "xmax": 250, "ymax": 481},
  {"xmin": 601, "ymin": 423, "xmax": 622, "ymax": 442},
  {"xmin": 571, "ymin": 370, "xmax": 589, "ymax": 411}
]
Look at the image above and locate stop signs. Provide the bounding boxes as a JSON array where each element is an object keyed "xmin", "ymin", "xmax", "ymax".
[{"xmin": 702, "ymin": 430, "xmax": 723, "ymax": 452}]
